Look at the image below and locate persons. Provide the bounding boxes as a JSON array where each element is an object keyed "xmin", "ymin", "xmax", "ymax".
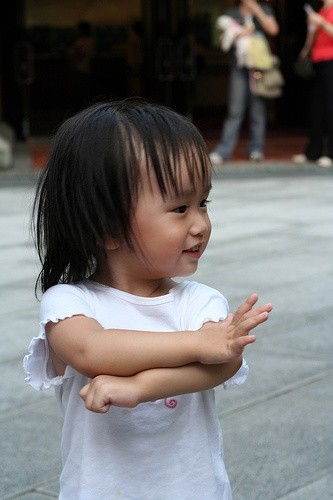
[
  {"xmin": 293, "ymin": 0, "xmax": 333, "ymax": 167},
  {"xmin": 22, "ymin": 98, "xmax": 273, "ymax": 500},
  {"xmin": 209, "ymin": 0, "xmax": 278, "ymax": 164}
]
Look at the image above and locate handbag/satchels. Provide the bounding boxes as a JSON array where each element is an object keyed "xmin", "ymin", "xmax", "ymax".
[
  {"xmin": 294, "ymin": 53, "xmax": 317, "ymax": 81},
  {"xmin": 247, "ymin": 67, "xmax": 287, "ymax": 99}
]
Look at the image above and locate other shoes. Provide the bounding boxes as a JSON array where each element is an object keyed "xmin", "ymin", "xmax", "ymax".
[
  {"xmin": 317, "ymin": 156, "xmax": 333, "ymax": 168},
  {"xmin": 293, "ymin": 152, "xmax": 307, "ymax": 163},
  {"xmin": 209, "ymin": 150, "xmax": 224, "ymax": 166},
  {"xmin": 250, "ymin": 150, "xmax": 265, "ymax": 162}
]
